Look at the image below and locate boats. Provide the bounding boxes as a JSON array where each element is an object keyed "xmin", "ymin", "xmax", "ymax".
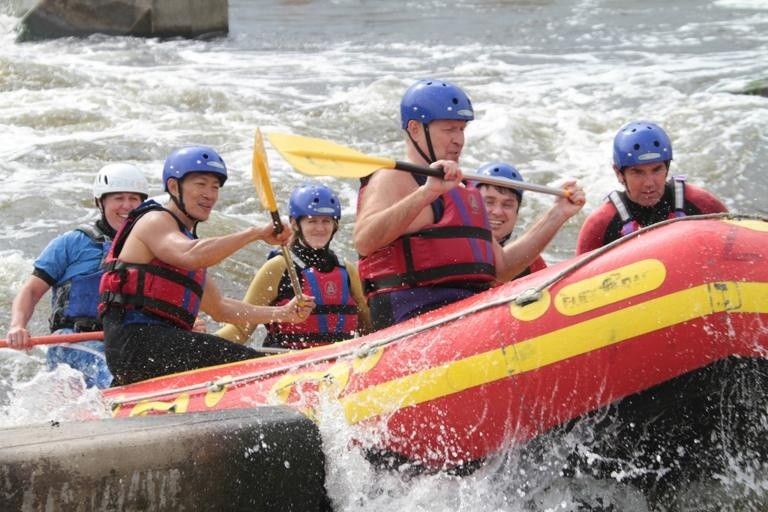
[{"xmin": 0, "ymin": 213, "xmax": 766, "ymax": 511}]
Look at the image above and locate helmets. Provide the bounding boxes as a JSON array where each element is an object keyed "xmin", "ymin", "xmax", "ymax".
[
  {"xmin": 613, "ymin": 119, "xmax": 672, "ymax": 171},
  {"xmin": 163, "ymin": 145, "xmax": 228, "ymax": 192},
  {"xmin": 92, "ymin": 162, "xmax": 149, "ymax": 206},
  {"xmin": 401, "ymin": 79, "xmax": 473, "ymax": 128},
  {"xmin": 288, "ymin": 184, "xmax": 341, "ymax": 224},
  {"xmin": 474, "ymin": 163, "xmax": 522, "ymax": 201}
]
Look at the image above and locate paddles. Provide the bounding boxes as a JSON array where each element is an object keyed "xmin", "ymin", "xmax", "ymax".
[
  {"xmin": 251, "ymin": 127, "xmax": 305, "ymax": 320},
  {"xmin": 267, "ymin": 130, "xmax": 571, "ymax": 198}
]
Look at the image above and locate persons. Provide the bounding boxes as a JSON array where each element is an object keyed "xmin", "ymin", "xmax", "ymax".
[
  {"xmin": 195, "ymin": 180, "xmax": 372, "ymax": 351},
  {"xmin": 470, "ymin": 163, "xmax": 548, "ymax": 278},
  {"xmin": 98, "ymin": 143, "xmax": 322, "ymax": 380},
  {"xmin": 3, "ymin": 159, "xmax": 153, "ymax": 396},
  {"xmin": 572, "ymin": 118, "xmax": 732, "ymax": 250},
  {"xmin": 347, "ymin": 77, "xmax": 590, "ymax": 332}
]
